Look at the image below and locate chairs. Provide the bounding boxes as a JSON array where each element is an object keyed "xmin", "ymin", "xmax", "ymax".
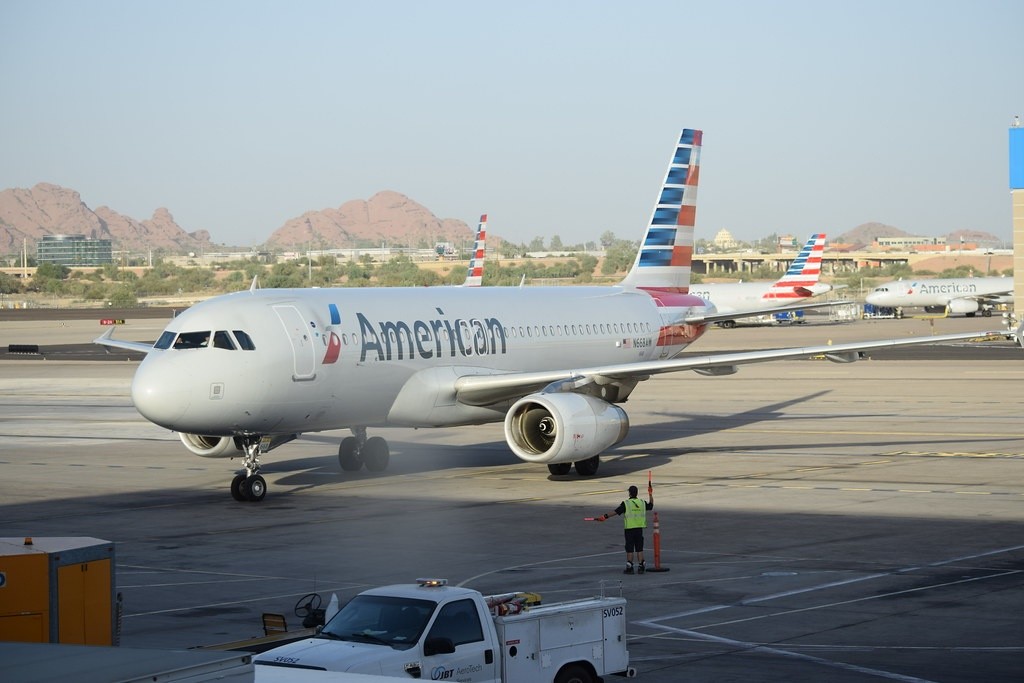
[
  {"xmin": 399, "ymin": 607, "xmax": 420, "ymax": 629},
  {"xmin": 262, "ymin": 612, "xmax": 288, "ymax": 637}
]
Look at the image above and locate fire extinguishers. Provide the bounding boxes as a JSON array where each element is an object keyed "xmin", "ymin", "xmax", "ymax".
[{"xmin": 494, "ymin": 602, "xmax": 526, "ymax": 616}]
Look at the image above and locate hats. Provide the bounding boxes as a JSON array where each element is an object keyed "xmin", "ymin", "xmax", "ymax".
[{"xmin": 627, "ymin": 486, "xmax": 637, "ymax": 496}]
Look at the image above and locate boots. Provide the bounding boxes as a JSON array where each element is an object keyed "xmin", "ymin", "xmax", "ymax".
[
  {"xmin": 624, "ymin": 561, "xmax": 634, "ymax": 574},
  {"xmin": 638, "ymin": 560, "xmax": 646, "ymax": 573}
]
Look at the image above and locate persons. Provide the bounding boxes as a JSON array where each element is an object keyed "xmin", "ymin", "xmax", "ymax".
[{"xmin": 598, "ymin": 485, "xmax": 654, "ymax": 574}]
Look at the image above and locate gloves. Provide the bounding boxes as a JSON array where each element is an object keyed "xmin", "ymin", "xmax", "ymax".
[
  {"xmin": 648, "ymin": 486, "xmax": 653, "ymax": 496},
  {"xmin": 598, "ymin": 514, "xmax": 608, "ymax": 521}
]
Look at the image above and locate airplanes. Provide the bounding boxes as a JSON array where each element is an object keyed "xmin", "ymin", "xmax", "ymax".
[
  {"xmin": 865, "ymin": 276, "xmax": 1014, "ymax": 318},
  {"xmin": 97, "ymin": 128, "xmax": 1024, "ymax": 503}
]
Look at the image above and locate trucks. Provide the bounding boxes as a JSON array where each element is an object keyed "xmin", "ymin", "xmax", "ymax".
[{"xmin": 251, "ymin": 578, "xmax": 636, "ymax": 683}]
